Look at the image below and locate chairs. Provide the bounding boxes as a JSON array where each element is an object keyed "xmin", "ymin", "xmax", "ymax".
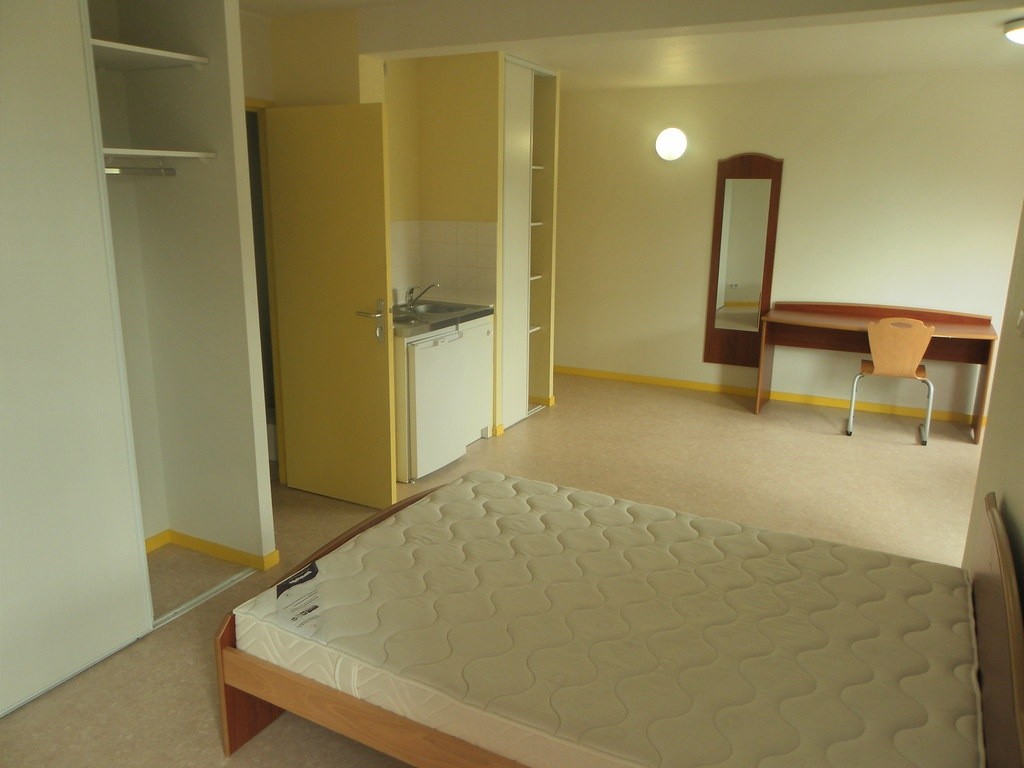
[{"xmin": 842, "ymin": 316, "xmax": 939, "ymax": 448}]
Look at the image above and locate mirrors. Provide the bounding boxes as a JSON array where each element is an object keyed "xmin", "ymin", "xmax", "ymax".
[{"xmin": 702, "ymin": 151, "xmax": 786, "ymax": 367}]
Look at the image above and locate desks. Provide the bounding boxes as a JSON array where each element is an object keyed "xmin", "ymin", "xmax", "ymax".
[{"xmin": 753, "ymin": 301, "xmax": 999, "ymax": 447}]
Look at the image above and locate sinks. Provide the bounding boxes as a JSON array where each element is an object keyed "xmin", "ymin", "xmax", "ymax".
[{"xmin": 392, "ymin": 301, "xmax": 469, "ymax": 327}]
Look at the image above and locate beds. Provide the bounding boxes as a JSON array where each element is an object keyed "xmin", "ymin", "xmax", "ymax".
[{"xmin": 208, "ymin": 457, "xmax": 1024, "ymax": 768}]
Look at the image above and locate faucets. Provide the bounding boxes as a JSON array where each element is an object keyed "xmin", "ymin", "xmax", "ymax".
[{"xmin": 405, "ymin": 282, "xmax": 440, "ymax": 311}]
